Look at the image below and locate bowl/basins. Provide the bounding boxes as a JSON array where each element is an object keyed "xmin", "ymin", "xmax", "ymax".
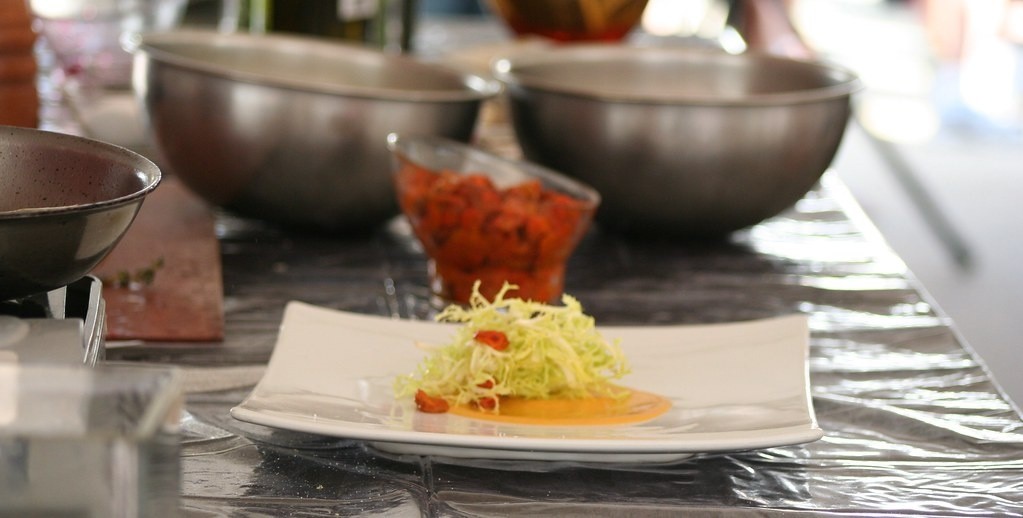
[
  {"xmin": 1, "ymin": 121, "xmax": 164, "ymax": 304},
  {"xmin": 120, "ymin": 29, "xmax": 502, "ymax": 239},
  {"xmin": 492, "ymin": 45, "xmax": 862, "ymax": 239}
]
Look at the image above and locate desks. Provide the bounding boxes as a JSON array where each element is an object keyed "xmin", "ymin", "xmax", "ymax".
[{"xmin": 107, "ymin": 174, "xmax": 1023, "ymax": 518}]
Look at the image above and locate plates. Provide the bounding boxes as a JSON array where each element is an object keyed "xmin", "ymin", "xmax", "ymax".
[{"xmin": 231, "ymin": 300, "xmax": 823, "ymax": 467}]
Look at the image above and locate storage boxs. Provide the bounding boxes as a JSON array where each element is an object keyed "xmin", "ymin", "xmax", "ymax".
[{"xmin": 0, "ymin": 357, "xmax": 187, "ymax": 518}]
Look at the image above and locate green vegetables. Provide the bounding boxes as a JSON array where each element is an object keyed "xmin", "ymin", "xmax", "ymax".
[{"xmin": 395, "ymin": 282, "xmax": 634, "ymax": 416}]
